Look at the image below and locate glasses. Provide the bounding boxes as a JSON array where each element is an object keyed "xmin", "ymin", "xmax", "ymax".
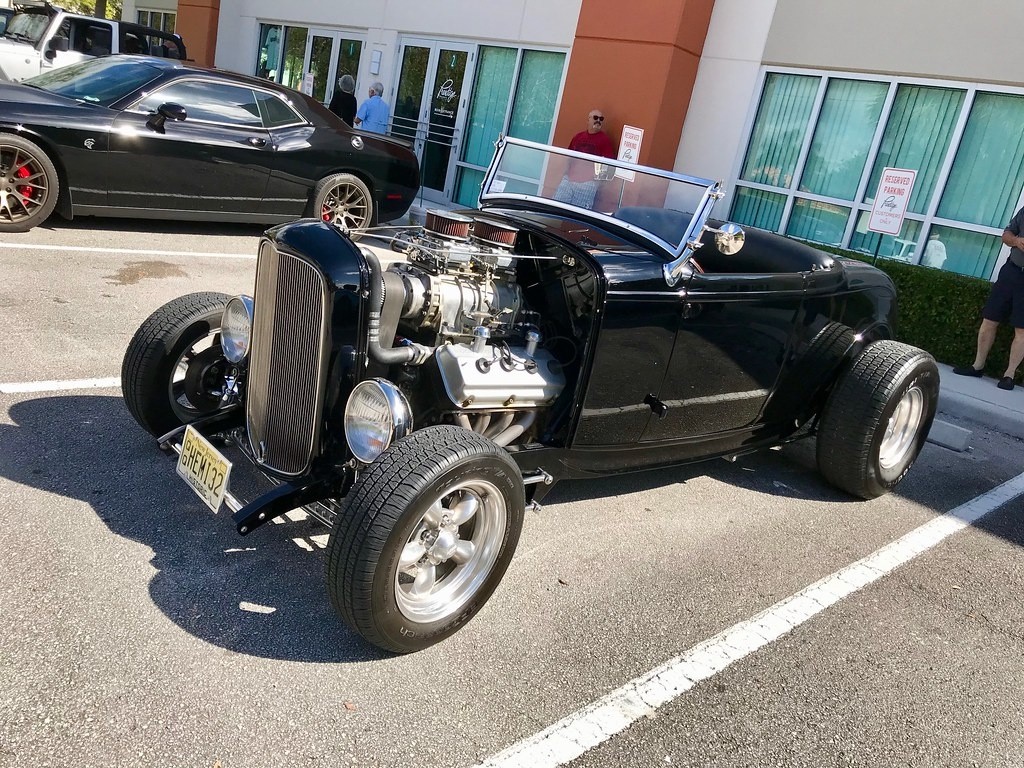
[{"xmin": 589, "ymin": 115, "xmax": 606, "ymax": 122}]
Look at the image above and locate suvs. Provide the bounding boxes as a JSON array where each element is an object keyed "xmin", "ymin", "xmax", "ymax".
[{"xmin": 0, "ymin": 0, "xmax": 187, "ymax": 82}]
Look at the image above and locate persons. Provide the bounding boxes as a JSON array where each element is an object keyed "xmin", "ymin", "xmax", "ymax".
[
  {"xmin": 552, "ymin": 109, "xmax": 618, "ymax": 211},
  {"xmin": 922, "ymin": 227, "xmax": 948, "ymax": 270},
  {"xmin": 158, "ymin": 34, "xmax": 182, "ymax": 59},
  {"xmin": 953, "ymin": 203, "xmax": 1024, "ymax": 391},
  {"xmin": 354, "ymin": 83, "xmax": 390, "ymax": 135},
  {"xmin": 328, "ymin": 75, "xmax": 358, "ymax": 129}
]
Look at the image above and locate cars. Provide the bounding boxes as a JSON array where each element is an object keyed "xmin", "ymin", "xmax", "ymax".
[
  {"xmin": 1, "ymin": 53, "xmax": 421, "ymax": 241},
  {"xmin": 732, "ymin": 193, "xmax": 918, "ymax": 262},
  {"xmin": 119, "ymin": 132, "xmax": 942, "ymax": 654}
]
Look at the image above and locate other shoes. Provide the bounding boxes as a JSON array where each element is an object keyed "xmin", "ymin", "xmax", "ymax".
[
  {"xmin": 953, "ymin": 364, "xmax": 985, "ymax": 378},
  {"xmin": 998, "ymin": 375, "xmax": 1014, "ymax": 390}
]
